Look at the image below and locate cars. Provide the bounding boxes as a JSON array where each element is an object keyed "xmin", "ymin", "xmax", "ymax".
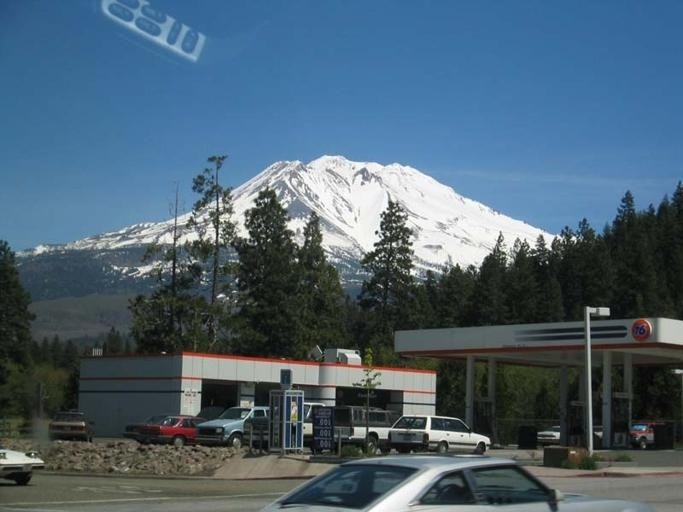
[
  {"xmin": 0, "ymin": 446, "xmax": 48, "ymax": 486},
  {"xmin": 257, "ymin": 451, "xmax": 660, "ymax": 512}
]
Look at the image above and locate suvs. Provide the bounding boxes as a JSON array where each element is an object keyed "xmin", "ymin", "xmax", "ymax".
[{"xmin": 48, "ymin": 408, "xmax": 96, "ymax": 442}]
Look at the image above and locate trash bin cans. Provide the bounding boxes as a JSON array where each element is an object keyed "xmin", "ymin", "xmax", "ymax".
[
  {"xmin": 518, "ymin": 427, "xmax": 537, "ymax": 449},
  {"xmin": 655, "ymin": 427, "xmax": 673, "ymax": 450}
]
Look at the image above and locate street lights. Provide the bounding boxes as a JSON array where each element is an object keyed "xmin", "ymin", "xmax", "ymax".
[{"xmin": 584, "ymin": 304, "xmax": 612, "ymax": 455}]
